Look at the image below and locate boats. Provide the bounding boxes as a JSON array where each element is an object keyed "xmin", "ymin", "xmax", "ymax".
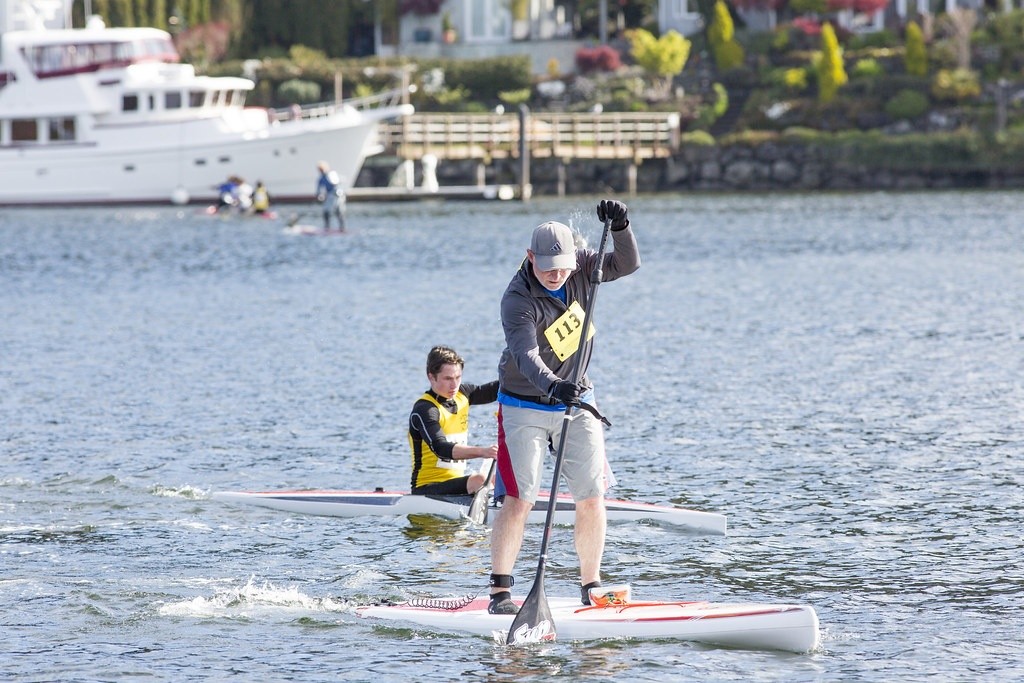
[{"xmin": 1, "ymin": 0, "xmax": 419, "ymax": 206}]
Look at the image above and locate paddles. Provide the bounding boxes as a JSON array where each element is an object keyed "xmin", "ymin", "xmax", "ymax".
[
  {"xmin": 505, "ymin": 212, "xmax": 613, "ymax": 644},
  {"xmin": 466, "ymin": 458, "xmax": 496, "ymax": 527}
]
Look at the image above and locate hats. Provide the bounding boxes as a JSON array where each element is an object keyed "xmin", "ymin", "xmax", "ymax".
[{"xmin": 532, "ymin": 221, "xmax": 576, "ymax": 272}]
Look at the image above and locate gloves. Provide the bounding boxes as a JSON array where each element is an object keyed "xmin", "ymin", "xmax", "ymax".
[
  {"xmin": 597, "ymin": 200, "xmax": 628, "ymax": 231},
  {"xmin": 546, "ymin": 379, "xmax": 587, "ymax": 407}
]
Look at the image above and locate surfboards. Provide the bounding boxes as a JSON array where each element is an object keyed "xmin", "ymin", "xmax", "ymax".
[
  {"xmin": 228, "ymin": 485, "xmax": 728, "ymax": 536},
  {"xmin": 347, "ymin": 592, "xmax": 821, "ymax": 653}
]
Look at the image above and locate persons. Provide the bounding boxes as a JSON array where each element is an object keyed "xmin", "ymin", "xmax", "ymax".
[
  {"xmin": 488, "ymin": 200, "xmax": 641, "ymax": 614},
  {"xmin": 209, "ymin": 177, "xmax": 275, "ymax": 216},
  {"xmin": 316, "ymin": 163, "xmax": 347, "ymax": 232},
  {"xmin": 408, "ymin": 347, "xmax": 499, "ymax": 494}
]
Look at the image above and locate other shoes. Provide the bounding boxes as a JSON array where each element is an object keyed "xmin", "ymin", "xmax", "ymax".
[
  {"xmin": 581, "ymin": 582, "xmax": 603, "ymax": 605},
  {"xmin": 488, "ymin": 591, "xmax": 521, "ymax": 614}
]
